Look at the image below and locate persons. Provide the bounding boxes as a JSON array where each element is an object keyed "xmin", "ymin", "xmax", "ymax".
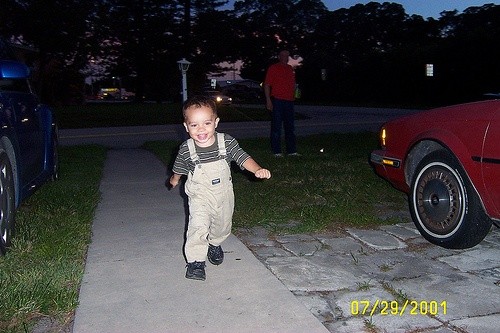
[
  {"xmin": 264, "ymin": 48, "xmax": 302, "ymax": 158},
  {"xmin": 170, "ymin": 96, "xmax": 271, "ymax": 280}
]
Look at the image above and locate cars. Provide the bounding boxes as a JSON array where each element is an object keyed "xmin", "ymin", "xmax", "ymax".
[
  {"xmin": 1, "ymin": 43, "xmax": 61, "ymax": 255},
  {"xmin": 196, "ymin": 90, "xmax": 232, "ymax": 106},
  {"xmin": 369, "ymin": 100, "xmax": 500, "ymax": 250}
]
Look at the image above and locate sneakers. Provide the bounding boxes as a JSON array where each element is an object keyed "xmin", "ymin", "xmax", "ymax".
[
  {"xmin": 207, "ymin": 244, "xmax": 224, "ymax": 265},
  {"xmin": 185, "ymin": 262, "xmax": 205, "ymax": 281}
]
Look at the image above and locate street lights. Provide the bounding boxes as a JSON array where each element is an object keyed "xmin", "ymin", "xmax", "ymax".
[{"xmin": 177, "ymin": 59, "xmax": 191, "ymax": 101}]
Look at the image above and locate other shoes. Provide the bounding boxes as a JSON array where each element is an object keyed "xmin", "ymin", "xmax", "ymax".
[
  {"xmin": 274, "ymin": 154, "xmax": 283, "ymax": 157},
  {"xmin": 288, "ymin": 152, "xmax": 303, "ymax": 156}
]
